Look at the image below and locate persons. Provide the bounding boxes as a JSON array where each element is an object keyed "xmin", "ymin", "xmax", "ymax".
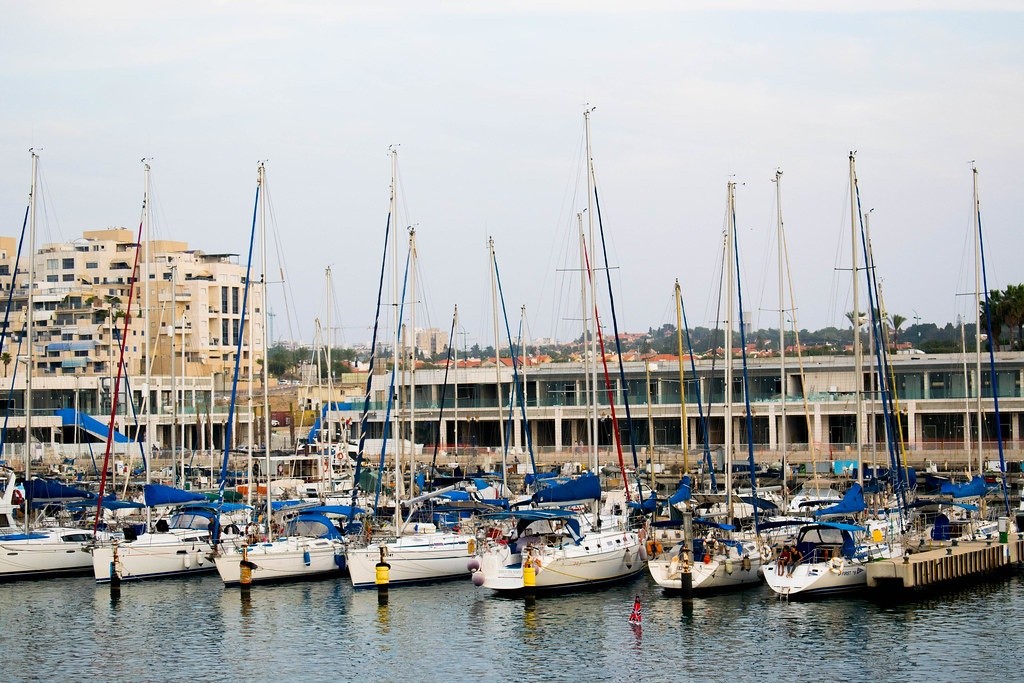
[
  {"xmin": 252, "ymin": 460, "xmax": 261, "ymax": 477},
  {"xmin": 207, "ymin": 516, "xmax": 223, "ymax": 543},
  {"xmin": 152, "ymin": 443, "xmax": 160, "ymax": 458},
  {"xmin": 777, "ymin": 545, "xmax": 790, "ymax": 576},
  {"xmin": 513, "ymin": 455, "xmax": 520, "ymax": 464},
  {"xmin": 786, "ymin": 545, "xmax": 803, "ymax": 577}
]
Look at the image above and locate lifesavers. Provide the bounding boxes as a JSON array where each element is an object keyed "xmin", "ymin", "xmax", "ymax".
[
  {"xmin": 829, "ymin": 557, "xmax": 844, "ymax": 574},
  {"xmin": 12, "ymin": 488, "xmax": 21, "ymax": 504},
  {"xmin": 760, "ymin": 545, "xmax": 772, "ymax": 560},
  {"xmin": 246, "ymin": 522, "xmax": 260, "ymax": 536},
  {"xmin": 468, "ymin": 538, "xmax": 475, "ymax": 554},
  {"xmin": 703, "ymin": 538, "xmax": 719, "ymax": 552},
  {"xmin": 645, "ymin": 539, "xmax": 662, "ymax": 555},
  {"xmin": 337, "ymin": 453, "xmax": 343, "ymax": 460},
  {"xmin": 277, "ymin": 464, "xmax": 284, "ymax": 474}
]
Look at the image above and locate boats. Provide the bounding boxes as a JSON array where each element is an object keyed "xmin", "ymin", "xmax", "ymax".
[{"xmin": 0, "ymin": 106, "xmax": 1024, "ymax": 598}]
